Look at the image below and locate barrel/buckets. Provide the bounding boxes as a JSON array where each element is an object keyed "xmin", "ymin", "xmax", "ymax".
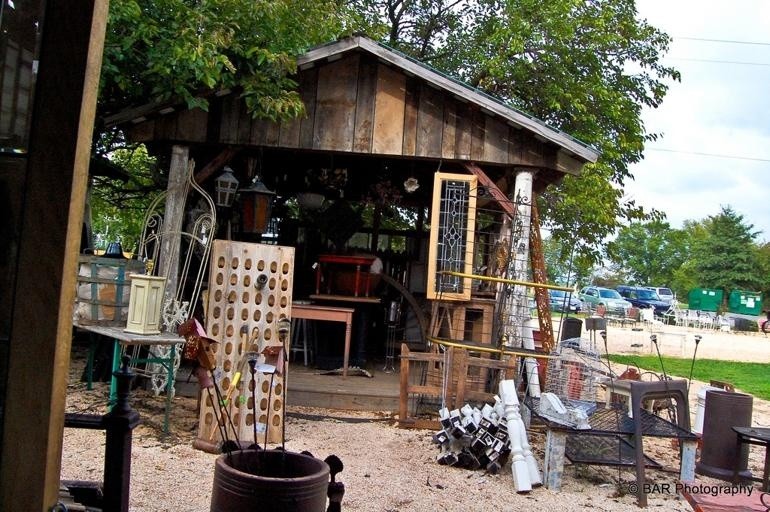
[{"xmin": 695, "ymin": 386, "xmax": 726, "ymax": 434}]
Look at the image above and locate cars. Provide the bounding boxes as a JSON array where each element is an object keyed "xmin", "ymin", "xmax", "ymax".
[{"xmin": 534, "ymin": 286, "xmax": 582, "ymax": 312}]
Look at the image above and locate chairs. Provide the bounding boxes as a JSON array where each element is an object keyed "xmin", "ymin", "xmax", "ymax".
[{"xmin": 587, "ymin": 300, "xmax": 730, "ymax": 335}]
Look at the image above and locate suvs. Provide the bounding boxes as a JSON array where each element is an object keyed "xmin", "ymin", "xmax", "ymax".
[
  {"xmin": 576, "ymin": 285, "xmax": 633, "ymax": 316},
  {"xmin": 616, "ymin": 284, "xmax": 671, "ymax": 318}
]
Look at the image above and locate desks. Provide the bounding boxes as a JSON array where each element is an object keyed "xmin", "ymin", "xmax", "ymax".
[
  {"xmin": 317, "ymin": 254, "xmax": 376, "ymax": 297},
  {"xmin": 518, "ymin": 395, "xmax": 701, "ymax": 501},
  {"xmin": 70, "ymin": 321, "xmax": 186, "ymax": 434},
  {"xmin": 309, "ymin": 294, "xmax": 381, "ymax": 370},
  {"xmin": 289, "ymin": 305, "xmax": 356, "ymax": 377},
  {"xmin": 730, "ymin": 425, "xmax": 770, "ymax": 492}
]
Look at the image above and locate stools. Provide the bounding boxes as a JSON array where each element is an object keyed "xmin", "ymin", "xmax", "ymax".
[{"xmin": 290, "ymin": 300, "xmax": 313, "ymax": 366}]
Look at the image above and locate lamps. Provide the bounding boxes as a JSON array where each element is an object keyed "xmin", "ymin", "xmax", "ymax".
[
  {"xmin": 215, "ymin": 166, "xmax": 239, "ymax": 208},
  {"xmin": 238, "ymin": 178, "xmax": 277, "ymax": 233}
]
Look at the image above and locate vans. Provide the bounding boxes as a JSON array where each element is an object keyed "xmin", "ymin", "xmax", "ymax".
[{"xmin": 647, "ymin": 285, "xmax": 677, "ymax": 305}]
